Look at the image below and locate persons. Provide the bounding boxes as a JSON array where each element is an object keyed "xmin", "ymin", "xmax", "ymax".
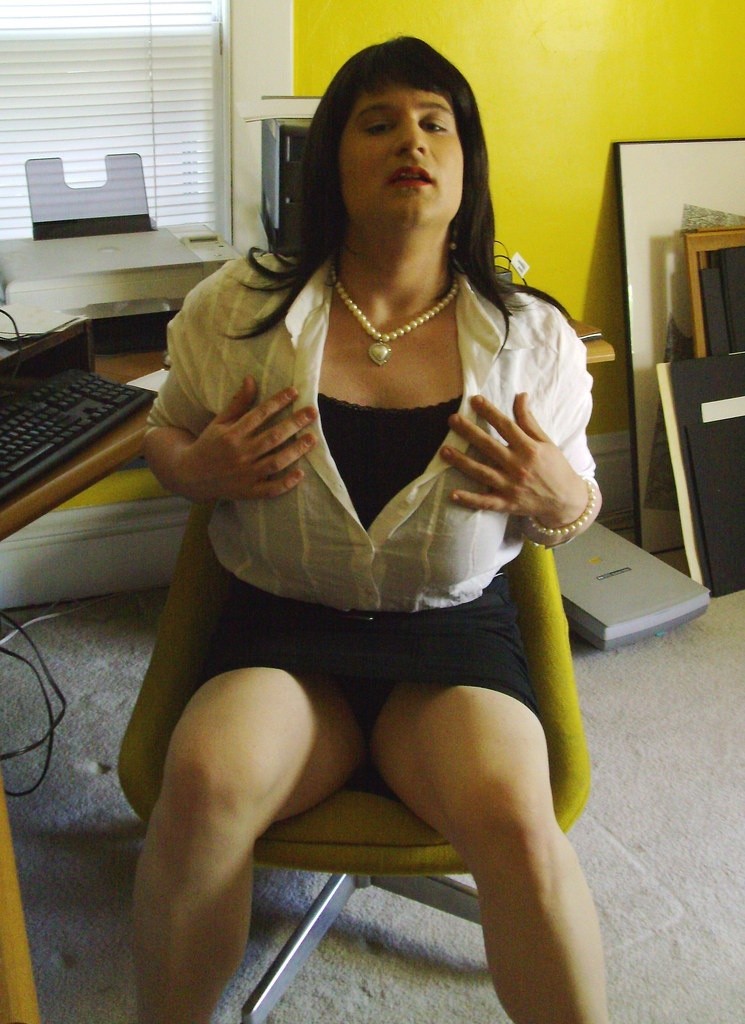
[{"xmin": 129, "ymin": 36, "xmax": 606, "ymax": 1024}]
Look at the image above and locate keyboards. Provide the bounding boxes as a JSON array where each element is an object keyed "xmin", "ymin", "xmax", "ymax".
[{"xmin": 0, "ymin": 372, "xmax": 158, "ymax": 502}]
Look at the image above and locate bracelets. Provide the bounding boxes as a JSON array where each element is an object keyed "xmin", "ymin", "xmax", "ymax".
[{"xmin": 529, "ymin": 479, "xmax": 597, "ymax": 538}]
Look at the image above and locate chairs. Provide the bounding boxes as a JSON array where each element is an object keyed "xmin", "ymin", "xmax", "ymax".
[{"xmin": 116, "ymin": 498, "xmax": 592, "ymax": 1023}]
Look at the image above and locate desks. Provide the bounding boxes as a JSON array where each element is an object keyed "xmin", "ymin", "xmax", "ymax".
[{"xmin": 0, "ymin": 316, "xmax": 616, "ymax": 1024}]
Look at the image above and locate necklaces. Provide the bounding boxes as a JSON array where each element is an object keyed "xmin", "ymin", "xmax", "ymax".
[{"xmin": 330, "ymin": 259, "xmax": 459, "ymax": 367}]
